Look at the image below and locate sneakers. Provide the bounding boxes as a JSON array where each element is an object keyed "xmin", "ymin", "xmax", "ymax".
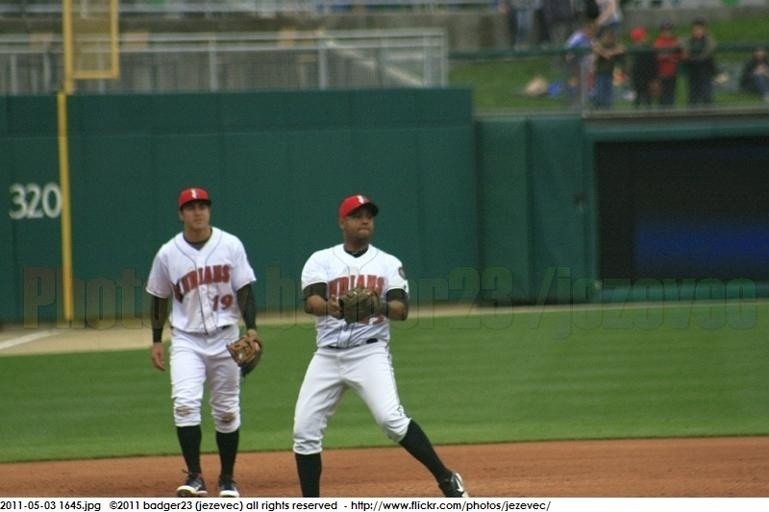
[
  {"xmin": 438, "ymin": 469, "xmax": 471, "ymax": 498},
  {"xmin": 215, "ymin": 474, "xmax": 243, "ymax": 498},
  {"xmin": 173, "ymin": 471, "xmax": 210, "ymax": 497}
]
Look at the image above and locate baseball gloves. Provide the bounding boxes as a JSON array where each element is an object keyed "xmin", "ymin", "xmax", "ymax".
[
  {"xmin": 226, "ymin": 335, "xmax": 262, "ymax": 377},
  {"xmin": 339, "ymin": 289, "xmax": 382, "ymax": 324}
]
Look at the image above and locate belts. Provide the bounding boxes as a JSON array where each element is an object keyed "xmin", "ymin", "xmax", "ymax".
[
  {"xmin": 325, "ymin": 337, "xmax": 380, "ymax": 352},
  {"xmin": 186, "ymin": 324, "xmax": 234, "ymax": 339}
]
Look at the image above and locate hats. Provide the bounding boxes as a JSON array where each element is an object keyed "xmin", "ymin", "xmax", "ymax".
[
  {"xmin": 337, "ymin": 192, "xmax": 380, "ymax": 221},
  {"xmin": 176, "ymin": 184, "xmax": 213, "ymax": 212}
]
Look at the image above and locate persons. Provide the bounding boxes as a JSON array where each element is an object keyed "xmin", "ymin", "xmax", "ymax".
[
  {"xmin": 494, "ymin": 1, "xmax": 769, "ymax": 113},
  {"xmin": 291, "ymin": 194, "xmax": 468, "ymax": 498},
  {"xmin": 145, "ymin": 188, "xmax": 264, "ymax": 497}
]
